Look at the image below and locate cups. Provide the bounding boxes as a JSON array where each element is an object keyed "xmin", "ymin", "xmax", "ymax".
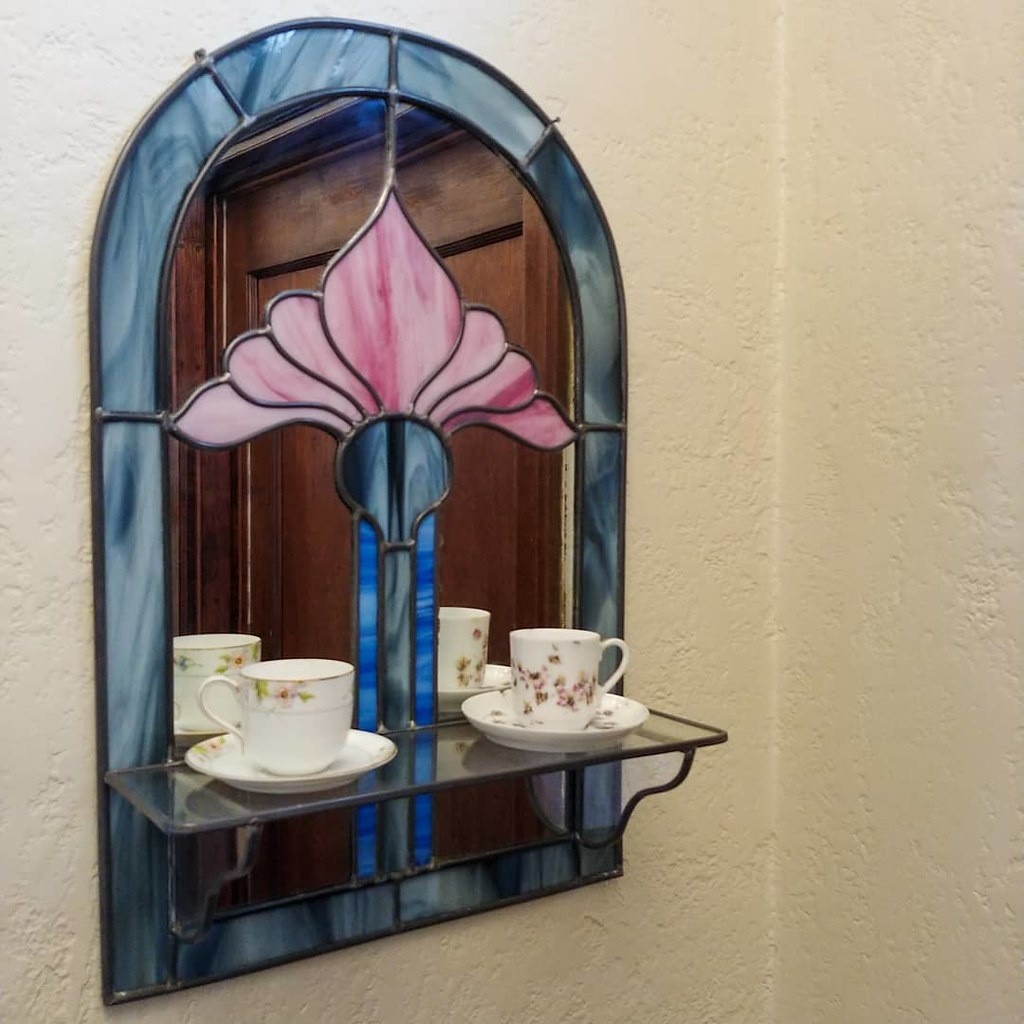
[
  {"xmin": 509, "ymin": 627, "xmax": 630, "ymax": 731},
  {"xmin": 172, "ymin": 634, "xmax": 261, "ymax": 733},
  {"xmin": 195, "ymin": 657, "xmax": 355, "ymax": 775},
  {"xmin": 436, "ymin": 606, "xmax": 492, "ymax": 691}
]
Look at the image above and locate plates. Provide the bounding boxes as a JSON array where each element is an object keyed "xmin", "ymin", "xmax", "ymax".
[
  {"xmin": 438, "ymin": 664, "xmax": 511, "ymax": 714},
  {"xmin": 184, "ymin": 728, "xmax": 398, "ymax": 797},
  {"xmin": 460, "ymin": 686, "xmax": 651, "ymax": 754}
]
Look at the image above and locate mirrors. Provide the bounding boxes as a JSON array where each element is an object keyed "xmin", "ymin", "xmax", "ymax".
[{"xmin": 85, "ymin": 21, "xmax": 728, "ymax": 1007}]
